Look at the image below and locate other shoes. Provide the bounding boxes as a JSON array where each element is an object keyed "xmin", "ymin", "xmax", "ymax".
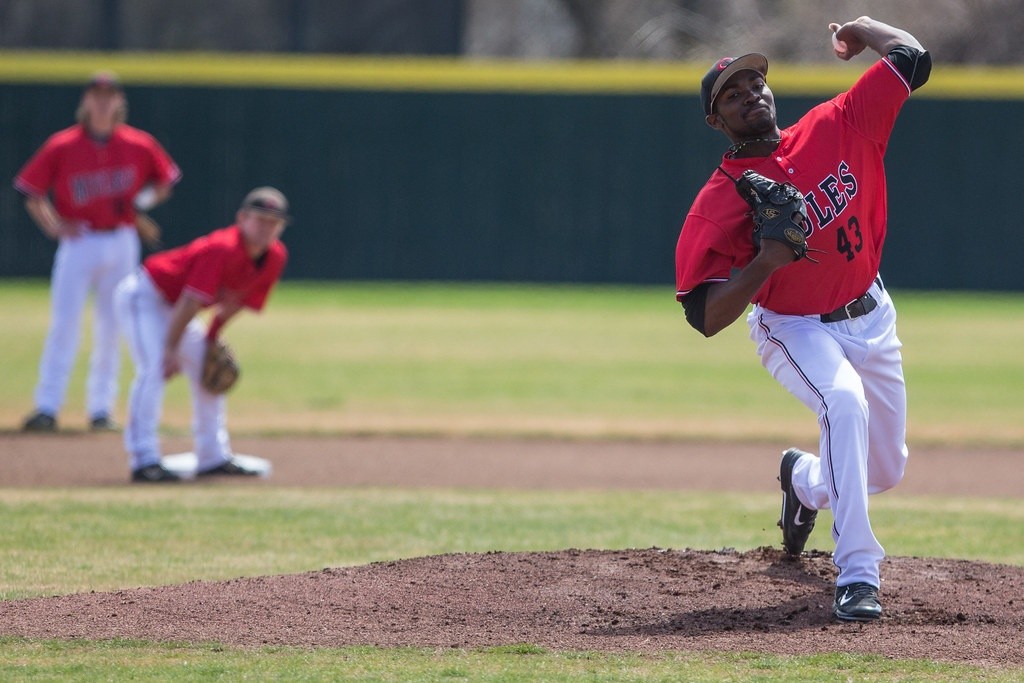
[
  {"xmin": 22, "ymin": 413, "xmax": 54, "ymax": 432},
  {"xmin": 197, "ymin": 458, "xmax": 257, "ymax": 477},
  {"xmin": 90, "ymin": 416, "xmax": 116, "ymax": 432},
  {"xmin": 131, "ymin": 464, "xmax": 180, "ymax": 482}
]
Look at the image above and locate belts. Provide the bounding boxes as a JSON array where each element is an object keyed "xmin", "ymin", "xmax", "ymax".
[{"xmin": 820, "ymin": 278, "xmax": 882, "ymax": 324}]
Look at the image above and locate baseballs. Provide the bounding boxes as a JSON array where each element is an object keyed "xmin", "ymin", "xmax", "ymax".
[{"xmin": 831, "ymin": 27, "xmax": 846, "ymax": 53}]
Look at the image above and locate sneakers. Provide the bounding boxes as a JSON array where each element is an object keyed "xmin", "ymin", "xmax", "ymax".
[
  {"xmin": 833, "ymin": 582, "xmax": 882, "ymax": 621},
  {"xmin": 777, "ymin": 447, "xmax": 818, "ymax": 558}
]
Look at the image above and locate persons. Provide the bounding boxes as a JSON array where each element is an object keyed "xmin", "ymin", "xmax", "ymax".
[
  {"xmin": 114, "ymin": 186, "xmax": 288, "ymax": 484},
  {"xmin": 675, "ymin": 16, "xmax": 932, "ymax": 621},
  {"xmin": 13, "ymin": 78, "xmax": 182, "ymax": 432}
]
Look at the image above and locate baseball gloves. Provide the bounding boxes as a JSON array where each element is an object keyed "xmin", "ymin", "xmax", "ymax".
[
  {"xmin": 197, "ymin": 341, "xmax": 239, "ymax": 395},
  {"xmin": 736, "ymin": 169, "xmax": 811, "ymax": 259}
]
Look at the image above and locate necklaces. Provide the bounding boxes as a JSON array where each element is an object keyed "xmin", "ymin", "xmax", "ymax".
[{"xmin": 728, "ymin": 138, "xmax": 782, "ymax": 158}]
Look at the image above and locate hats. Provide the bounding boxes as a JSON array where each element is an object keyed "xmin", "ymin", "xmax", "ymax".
[
  {"xmin": 243, "ymin": 186, "xmax": 288, "ymax": 218},
  {"xmin": 701, "ymin": 51, "xmax": 768, "ymax": 118},
  {"xmin": 87, "ymin": 73, "xmax": 120, "ymax": 91}
]
